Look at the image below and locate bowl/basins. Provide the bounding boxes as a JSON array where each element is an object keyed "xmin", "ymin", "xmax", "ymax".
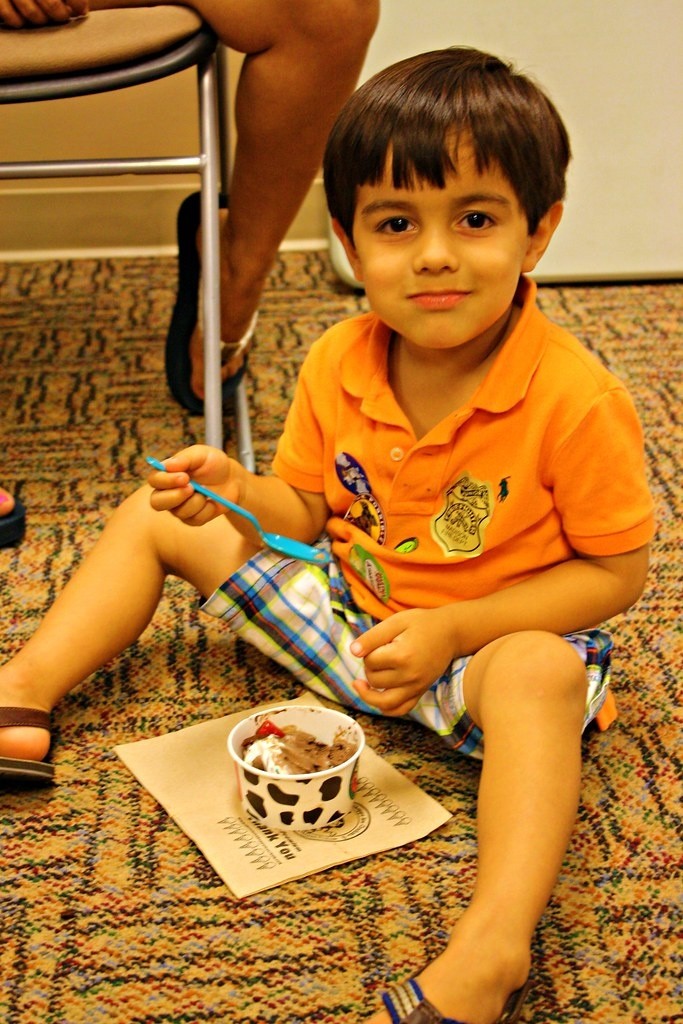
[{"xmin": 228, "ymin": 705, "xmax": 365, "ymax": 830}]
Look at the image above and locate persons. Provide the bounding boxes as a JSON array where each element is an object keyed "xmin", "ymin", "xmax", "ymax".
[
  {"xmin": 0, "ymin": 0, "xmax": 382, "ymax": 550},
  {"xmin": 0, "ymin": 46, "xmax": 659, "ymax": 1024}
]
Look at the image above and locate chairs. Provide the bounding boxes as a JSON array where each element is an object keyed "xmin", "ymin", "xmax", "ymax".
[{"xmin": 0, "ymin": 5, "xmax": 259, "ymax": 472}]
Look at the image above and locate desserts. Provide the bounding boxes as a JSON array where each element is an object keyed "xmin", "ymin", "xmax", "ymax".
[{"xmin": 243, "ymin": 723, "xmax": 354, "ymax": 774}]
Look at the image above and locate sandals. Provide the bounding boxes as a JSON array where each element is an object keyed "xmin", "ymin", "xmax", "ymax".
[
  {"xmin": 382, "ymin": 949, "xmax": 531, "ymax": 1024},
  {"xmin": 0, "ymin": 707, "xmax": 55, "ymax": 778}
]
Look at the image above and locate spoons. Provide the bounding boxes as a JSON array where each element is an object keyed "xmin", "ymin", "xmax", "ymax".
[{"xmin": 145, "ymin": 456, "xmax": 332, "ymax": 565}]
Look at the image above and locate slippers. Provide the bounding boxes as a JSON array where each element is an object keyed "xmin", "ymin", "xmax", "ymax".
[
  {"xmin": 0, "ymin": 496, "xmax": 25, "ymax": 547},
  {"xmin": 165, "ymin": 191, "xmax": 258, "ymax": 413}
]
